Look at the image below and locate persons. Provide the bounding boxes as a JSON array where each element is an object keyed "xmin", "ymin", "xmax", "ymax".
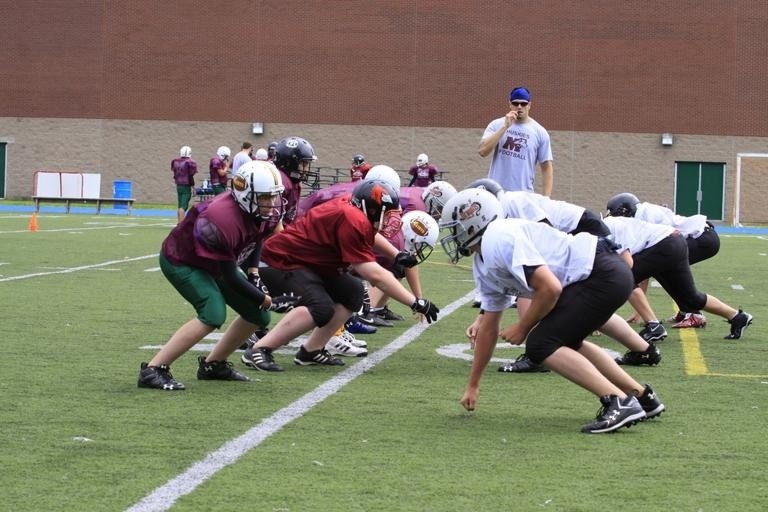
[
  {"xmin": 437, "ymin": 187, "xmax": 666, "ymax": 435},
  {"xmin": 136, "ymin": 160, "xmax": 288, "ymax": 391},
  {"xmin": 171, "ymin": 141, "xmax": 278, "ymax": 224},
  {"xmin": 438, "ymin": 178, "xmax": 752, "ymax": 372},
  {"xmin": 478, "ymin": 86, "xmax": 554, "ymax": 198},
  {"xmin": 237, "ymin": 136, "xmax": 458, "ymax": 372}
]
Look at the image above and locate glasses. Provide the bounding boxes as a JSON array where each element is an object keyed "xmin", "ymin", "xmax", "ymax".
[{"xmin": 510, "ymin": 102, "xmax": 530, "ymax": 107}]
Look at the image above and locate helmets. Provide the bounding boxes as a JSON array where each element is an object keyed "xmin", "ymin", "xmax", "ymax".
[
  {"xmin": 608, "ymin": 193, "xmax": 639, "ymax": 216},
  {"xmin": 181, "ymin": 146, "xmax": 192, "ymax": 158},
  {"xmin": 268, "ymin": 141, "xmax": 278, "ymax": 153},
  {"xmin": 256, "ymin": 149, "xmax": 268, "ymax": 160},
  {"xmin": 351, "ymin": 165, "xmax": 402, "ymax": 222},
  {"xmin": 424, "ymin": 182, "xmax": 456, "ymax": 216},
  {"xmin": 403, "ymin": 210, "xmax": 439, "ymax": 259},
  {"xmin": 467, "ymin": 178, "xmax": 502, "ymax": 193},
  {"xmin": 441, "ymin": 189, "xmax": 499, "ymax": 262},
  {"xmin": 217, "ymin": 146, "xmax": 231, "ymax": 160},
  {"xmin": 417, "ymin": 154, "xmax": 428, "ymax": 168},
  {"xmin": 233, "ymin": 161, "xmax": 282, "ymax": 217},
  {"xmin": 274, "ymin": 138, "xmax": 317, "ymax": 181},
  {"xmin": 352, "ymin": 155, "xmax": 365, "ymax": 164}
]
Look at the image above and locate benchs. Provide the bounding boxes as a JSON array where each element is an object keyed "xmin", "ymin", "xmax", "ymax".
[
  {"xmin": 192, "ymin": 164, "xmax": 452, "ymax": 205},
  {"xmin": 30, "ymin": 193, "xmax": 137, "ymax": 217}
]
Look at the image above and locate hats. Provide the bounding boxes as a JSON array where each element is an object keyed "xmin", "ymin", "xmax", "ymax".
[{"xmin": 509, "ymin": 88, "xmax": 531, "ymax": 102}]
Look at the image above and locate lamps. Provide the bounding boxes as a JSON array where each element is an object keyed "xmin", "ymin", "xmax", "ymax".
[
  {"xmin": 249, "ymin": 121, "xmax": 265, "ymax": 136},
  {"xmin": 659, "ymin": 132, "xmax": 676, "ymax": 146}
]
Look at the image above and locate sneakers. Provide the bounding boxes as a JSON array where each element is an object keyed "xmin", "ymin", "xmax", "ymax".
[
  {"xmin": 639, "ymin": 320, "xmax": 668, "ymax": 342},
  {"xmin": 293, "ymin": 345, "xmax": 346, "ymax": 366},
  {"xmin": 497, "ymin": 352, "xmax": 552, "ymax": 373},
  {"xmin": 667, "ymin": 312, "xmax": 707, "ymax": 328},
  {"xmin": 324, "ymin": 304, "xmax": 405, "ymax": 358},
  {"xmin": 723, "ymin": 309, "xmax": 754, "ymax": 340},
  {"xmin": 241, "ymin": 346, "xmax": 285, "ymax": 372},
  {"xmin": 614, "ymin": 341, "xmax": 662, "ymax": 367},
  {"xmin": 580, "ymin": 393, "xmax": 647, "ymax": 434},
  {"xmin": 237, "ymin": 327, "xmax": 269, "ymax": 350},
  {"xmin": 137, "ymin": 362, "xmax": 186, "ymax": 391},
  {"xmin": 637, "ymin": 383, "xmax": 667, "ymax": 419},
  {"xmin": 196, "ymin": 355, "xmax": 259, "ymax": 381},
  {"xmin": 472, "ymin": 292, "xmax": 518, "ymax": 307}
]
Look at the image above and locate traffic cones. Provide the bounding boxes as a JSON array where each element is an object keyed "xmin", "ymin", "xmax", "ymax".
[{"xmin": 29, "ymin": 212, "xmax": 38, "ymax": 232}]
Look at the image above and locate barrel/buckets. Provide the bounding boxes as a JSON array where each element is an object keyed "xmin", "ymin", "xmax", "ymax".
[{"xmin": 113, "ymin": 181, "xmax": 131, "ymax": 209}]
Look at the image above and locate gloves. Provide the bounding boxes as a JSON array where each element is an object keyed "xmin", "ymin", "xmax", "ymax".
[
  {"xmin": 247, "ymin": 271, "xmax": 270, "ymax": 313},
  {"xmin": 361, "ymin": 285, "xmax": 371, "ymax": 318},
  {"xmin": 263, "ymin": 292, "xmax": 302, "ymax": 314},
  {"xmin": 410, "ymin": 296, "xmax": 440, "ymax": 324}
]
